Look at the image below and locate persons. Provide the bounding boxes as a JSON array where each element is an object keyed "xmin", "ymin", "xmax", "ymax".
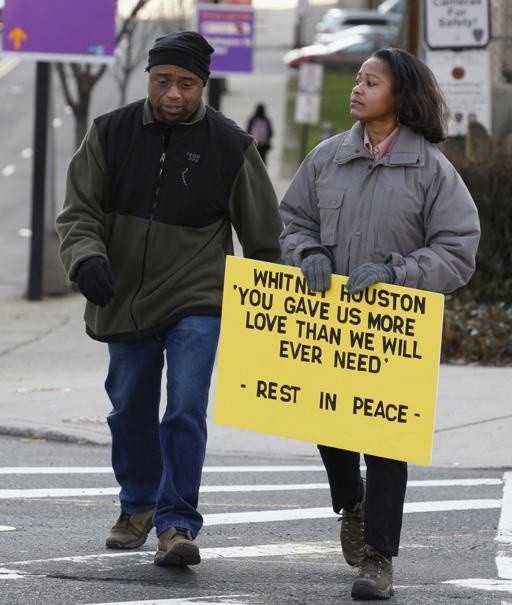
[
  {"xmin": 247, "ymin": 104, "xmax": 272, "ymax": 165},
  {"xmin": 54, "ymin": 31, "xmax": 285, "ymax": 567},
  {"xmin": 278, "ymin": 48, "xmax": 482, "ymax": 600}
]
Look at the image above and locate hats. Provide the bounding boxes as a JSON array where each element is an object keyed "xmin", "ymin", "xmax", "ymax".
[{"xmin": 144, "ymin": 31, "xmax": 217, "ymax": 86}]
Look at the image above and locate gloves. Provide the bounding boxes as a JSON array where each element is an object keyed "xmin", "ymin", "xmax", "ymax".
[
  {"xmin": 75, "ymin": 255, "xmax": 118, "ymax": 309},
  {"xmin": 301, "ymin": 254, "xmax": 332, "ymax": 294},
  {"xmin": 342, "ymin": 261, "xmax": 395, "ymax": 297}
]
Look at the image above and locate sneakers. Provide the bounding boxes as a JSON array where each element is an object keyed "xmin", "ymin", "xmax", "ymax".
[
  {"xmin": 104, "ymin": 499, "xmax": 159, "ymax": 551},
  {"xmin": 351, "ymin": 548, "xmax": 396, "ymax": 600},
  {"xmin": 338, "ymin": 475, "xmax": 368, "ymax": 569},
  {"xmin": 153, "ymin": 526, "xmax": 203, "ymax": 569}
]
